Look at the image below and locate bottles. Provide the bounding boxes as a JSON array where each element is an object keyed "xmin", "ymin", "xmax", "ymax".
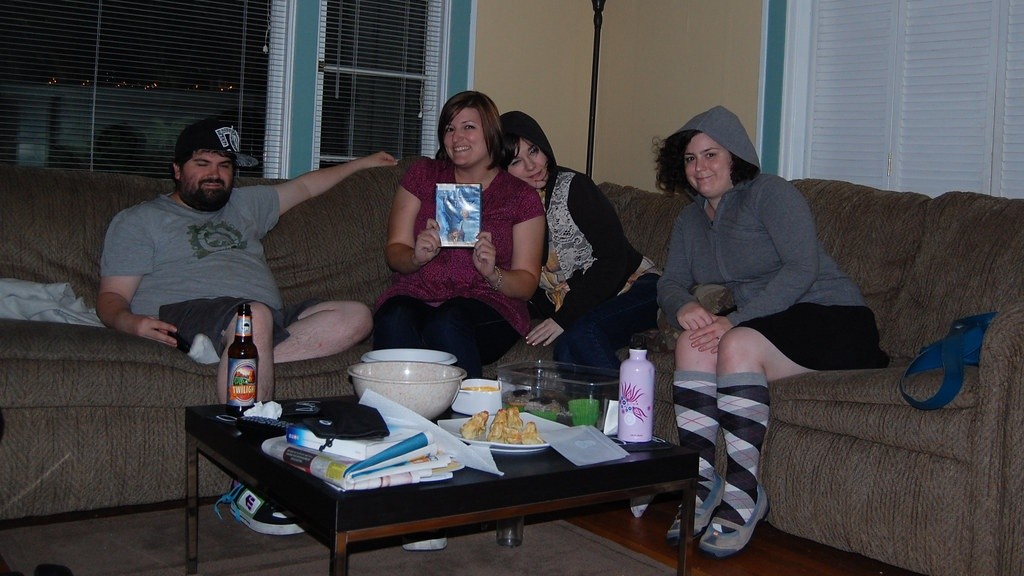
[
  {"xmin": 618, "ymin": 335, "xmax": 654, "ymax": 443},
  {"xmin": 227, "ymin": 304, "xmax": 258, "ymax": 416}
]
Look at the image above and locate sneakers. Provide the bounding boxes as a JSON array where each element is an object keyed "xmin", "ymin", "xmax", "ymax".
[{"xmin": 230, "ymin": 479, "xmax": 306, "ymax": 535}]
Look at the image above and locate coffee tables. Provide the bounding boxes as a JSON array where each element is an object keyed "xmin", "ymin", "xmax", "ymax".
[{"xmin": 184, "ymin": 395, "xmax": 700, "ymax": 576}]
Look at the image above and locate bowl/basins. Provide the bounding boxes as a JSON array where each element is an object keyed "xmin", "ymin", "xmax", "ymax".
[
  {"xmin": 452, "ymin": 379, "xmax": 502, "ymax": 416},
  {"xmin": 361, "ymin": 349, "xmax": 457, "ymax": 365},
  {"xmin": 347, "ymin": 361, "xmax": 467, "ymax": 420}
]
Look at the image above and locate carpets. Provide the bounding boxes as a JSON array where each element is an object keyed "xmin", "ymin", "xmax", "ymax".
[{"xmin": 0, "ymin": 497, "xmax": 675, "ymax": 576}]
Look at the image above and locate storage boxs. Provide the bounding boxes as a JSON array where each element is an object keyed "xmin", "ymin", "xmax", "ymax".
[{"xmin": 493, "ymin": 361, "xmax": 619, "ymax": 431}]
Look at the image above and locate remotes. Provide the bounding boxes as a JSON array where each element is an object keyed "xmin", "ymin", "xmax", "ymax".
[{"xmin": 240, "ymin": 415, "xmax": 292, "ymax": 433}]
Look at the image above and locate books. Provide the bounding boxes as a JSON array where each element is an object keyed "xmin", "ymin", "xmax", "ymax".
[{"xmin": 286, "ymin": 425, "xmax": 422, "ymax": 462}]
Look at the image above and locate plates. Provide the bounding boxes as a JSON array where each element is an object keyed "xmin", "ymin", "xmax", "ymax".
[{"xmin": 437, "ymin": 412, "xmax": 570, "ymax": 453}]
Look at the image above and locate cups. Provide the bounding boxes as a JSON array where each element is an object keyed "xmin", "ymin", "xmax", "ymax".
[
  {"xmin": 496, "ymin": 516, "xmax": 524, "ymax": 546},
  {"xmin": 568, "ymin": 399, "xmax": 600, "ymax": 426}
]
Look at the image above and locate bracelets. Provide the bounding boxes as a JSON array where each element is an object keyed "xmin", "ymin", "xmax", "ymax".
[{"xmin": 484, "ymin": 266, "xmax": 503, "ymax": 290}]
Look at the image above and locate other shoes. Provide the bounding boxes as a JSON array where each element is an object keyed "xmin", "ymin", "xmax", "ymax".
[
  {"xmin": 698, "ymin": 483, "xmax": 772, "ymax": 558},
  {"xmin": 668, "ymin": 473, "xmax": 725, "ymax": 544}
]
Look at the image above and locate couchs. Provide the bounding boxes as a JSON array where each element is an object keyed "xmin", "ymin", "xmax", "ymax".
[{"xmin": 0, "ymin": 152, "xmax": 1024, "ymax": 576}]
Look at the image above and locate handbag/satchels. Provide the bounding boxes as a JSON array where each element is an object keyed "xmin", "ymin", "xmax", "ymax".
[{"xmin": 901, "ymin": 311, "xmax": 1001, "ymax": 410}]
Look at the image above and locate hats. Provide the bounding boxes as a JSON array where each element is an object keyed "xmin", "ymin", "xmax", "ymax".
[{"xmin": 175, "ymin": 119, "xmax": 259, "ymax": 168}]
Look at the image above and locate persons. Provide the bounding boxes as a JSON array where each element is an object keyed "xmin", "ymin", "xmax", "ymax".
[
  {"xmin": 498, "ymin": 112, "xmax": 662, "ymax": 517},
  {"xmin": 656, "ymin": 107, "xmax": 880, "ymax": 559},
  {"xmin": 374, "ymin": 92, "xmax": 546, "ymax": 550},
  {"xmin": 97, "ymin": 122, "xmax": 398, "ymax": 534}
]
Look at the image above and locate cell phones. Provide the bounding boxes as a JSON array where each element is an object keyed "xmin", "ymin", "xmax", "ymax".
[{"xmin": 166, "ymin": 330, "xmax": 191, "ymax": 354}]
